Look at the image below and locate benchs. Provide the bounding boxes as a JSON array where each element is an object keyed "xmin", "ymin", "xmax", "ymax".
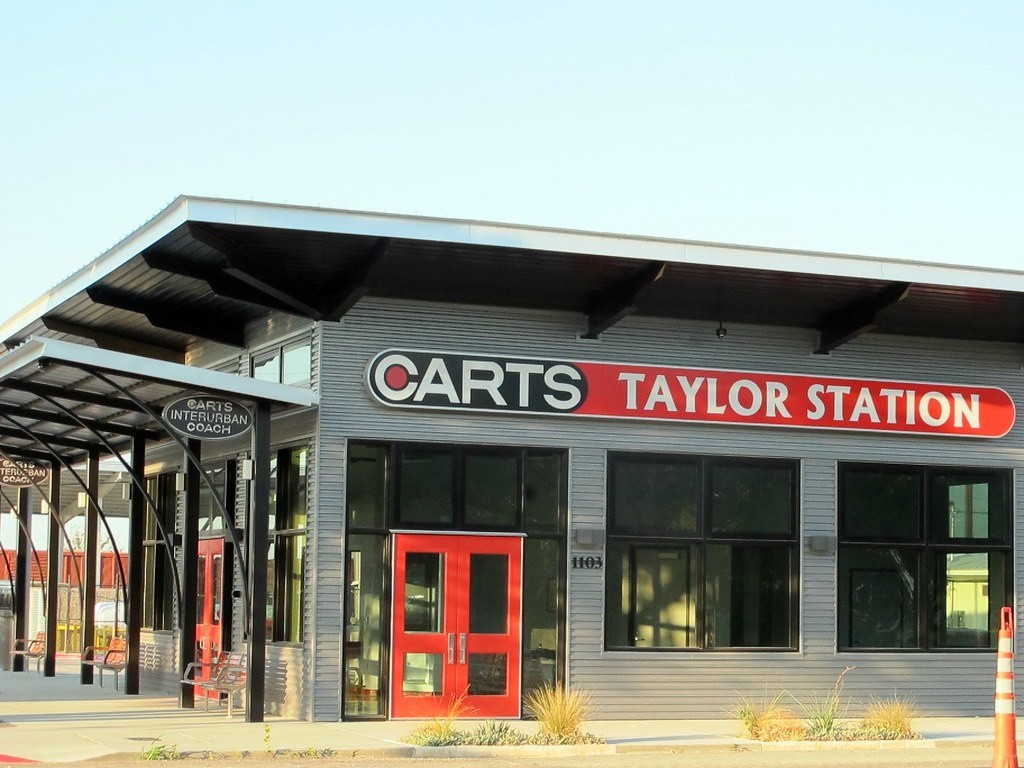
[
  {"xmin": 80, "ymin": 638, "xmax": 130, "ymax": 691},
  {"xmin": 181, "ymin": 651, "xmax": 250, "ymax": 719},
  {"xmin": 9, "ymin": 631, "xmax": 46, "ymax": 674}
]
